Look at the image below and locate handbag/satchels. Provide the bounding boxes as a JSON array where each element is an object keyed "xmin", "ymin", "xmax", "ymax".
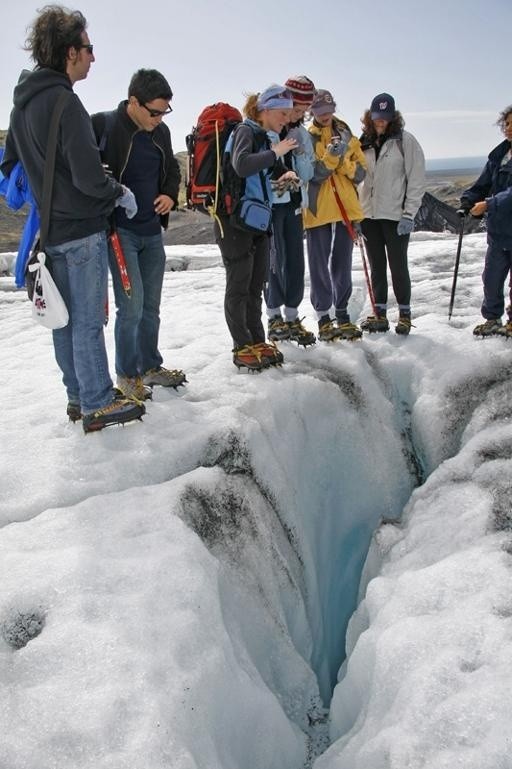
[
  {"xmin": 24, "ymin": 241, "xmax": 48, "ymax": 300},
  {"xmin": 232, "ymin": 199, "xmax": 273, "ymax": 233}
]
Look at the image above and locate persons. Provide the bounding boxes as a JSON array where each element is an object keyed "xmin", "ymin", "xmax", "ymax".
[
  {"xmin": 456, "ymin": 104, "xmax": 512, "ymax": 338},
  {"xmin": 0, "ymin": 5, "xmax": 146, "ymax": 435},
  {"xmin": 215, "ymin": 83, "xmax": 301, "ymax": 369},
  {"xmin": 266, "ymin": 74, "xmax": 318, "ymax": 350},
  {"xmin": 304, "ymin": 87, "xmax": 368, "ymax": 344},
  {"xmin": 354, "ymin": 92, "xmax": 427, "ymax": 336},
  {"xmin": 83, "ymin": 68, "xmax": 186, "ymax": 405}
]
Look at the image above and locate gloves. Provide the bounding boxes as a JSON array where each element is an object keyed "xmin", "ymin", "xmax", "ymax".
[
  {"xmin": 327, "ymin": 141, "xmax": 347, "ymax": 157},
  {"xmin": 286, "ymin": 128, "xmax": 306, "ymax": 155},
  {"xmin": 456, "ymin": 200, "xmax": 485, "ymax": 219},
  {"xmin": 396, "ymin": 218, "xmax": 414, "ymax": 235}
]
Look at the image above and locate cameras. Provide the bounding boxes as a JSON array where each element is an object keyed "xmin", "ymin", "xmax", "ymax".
[{"xmin": 330, "ymin": 135, "xmax": 341, "ymax": 148}]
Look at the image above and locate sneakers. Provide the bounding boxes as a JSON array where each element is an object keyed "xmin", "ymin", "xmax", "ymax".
[
  {"xmin": 496, "ymin": 321, "xmax": 512, "ymax": 336},
  {"xmin": 232, "ymin": 317, "xmax": 410, "ymax": 370},
  {"xmin": 67, "ymin": 367, "xmax": 186, "ymax": 430},
  {"xmin": 473, "ymin": 318, "xmax": 501, "ymax": 335}
]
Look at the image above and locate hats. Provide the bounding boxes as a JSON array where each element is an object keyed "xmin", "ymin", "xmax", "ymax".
[{"xmin": 258, "ymin": 75, "xmax": 395, "ymax": 120}]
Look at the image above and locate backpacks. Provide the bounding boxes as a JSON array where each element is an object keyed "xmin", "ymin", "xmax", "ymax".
[{"xmin": 185, "ymin": 102, "xmax": 259, "ymax": 216}]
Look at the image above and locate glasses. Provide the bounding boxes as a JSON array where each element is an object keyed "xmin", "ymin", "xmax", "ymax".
[
  {"xmin": 138, "ymin": 104, "xmax": 173, "ymax": 117},
  {"xmin": 77, "ymin": 44, "xmax": 94, "ymax": 56}
]
